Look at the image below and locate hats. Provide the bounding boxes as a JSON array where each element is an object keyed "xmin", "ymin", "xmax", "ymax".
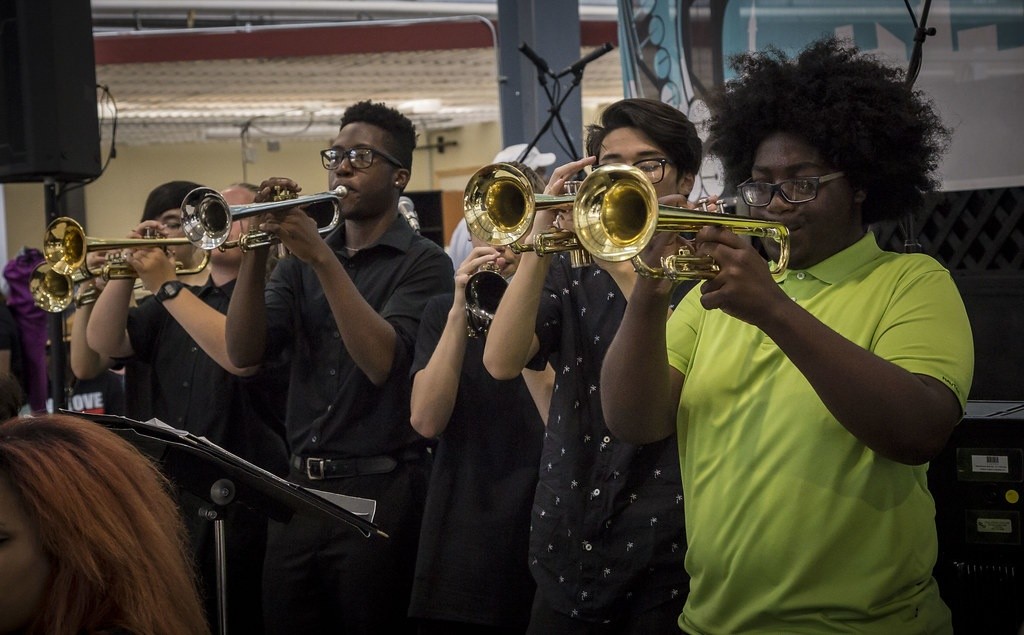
[
  {"xmin": 139, "ymin": 180, "xmax": 206, "ymax": 223},
  {"xmin": 492, "ymin": 143, "xmax": 556, "ymax": 172}
]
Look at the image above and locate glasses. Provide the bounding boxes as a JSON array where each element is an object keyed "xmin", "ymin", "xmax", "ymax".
[
  {"xmin": 736, "ymin": 171, "xmax": 841, "ymax": 208},
  {"xmin": 319, "ymin": 143, "xmax": 404, "ymax": 170},
  {"xmin": 591, "ymin": 155, "xmax": 675, "ymax": 185}
]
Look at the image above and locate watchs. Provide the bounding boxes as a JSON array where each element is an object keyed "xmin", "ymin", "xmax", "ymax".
[{"xmin": 154, "ymin": 280, "xmax": 191, "ymax": 303}]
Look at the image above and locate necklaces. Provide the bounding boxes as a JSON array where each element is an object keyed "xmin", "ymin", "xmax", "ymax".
[{"xmin": 345, "ymin": 246, "xmax": 358, "ymax": 252}]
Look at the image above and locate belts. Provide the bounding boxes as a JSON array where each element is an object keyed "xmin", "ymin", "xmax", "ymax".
[{"xmin": 288, "ymin": 454, "xmax": 415, "ymax": 479}]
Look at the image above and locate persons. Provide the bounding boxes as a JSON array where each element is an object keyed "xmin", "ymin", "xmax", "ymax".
[
  {"xmin": 599, "ymin": 24, "xmax": 977, "ymax": 635},
  {"xmin": 0, "ymin": 100, "xmax": 559, "ymax": 635},
  {"xmin": 482, "ymin": 96, "xmax": 769, "ymax": 635}
]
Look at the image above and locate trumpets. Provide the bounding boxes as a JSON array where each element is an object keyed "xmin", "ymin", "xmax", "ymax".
[
  {"xmin": 40, "ymin": 215, "xmax": 210, "ymax": 283},
  {"xmin": 571, "ymin": 160, "xmax": 792, "ymax": 286},
  {"xmin": 178, "ymin": 180, "xmax": 343, "ymax": 256},
  {"xmin": 464, "ymin": 245, "xmax": 510, "ymax": 321},
  {"xmin": 461, "ymin": 158, "xmax": 596, "ymax": 272},
  {"xmin": 28, "ymin": 259, "xmax": 104, "ymax": 315}
]
